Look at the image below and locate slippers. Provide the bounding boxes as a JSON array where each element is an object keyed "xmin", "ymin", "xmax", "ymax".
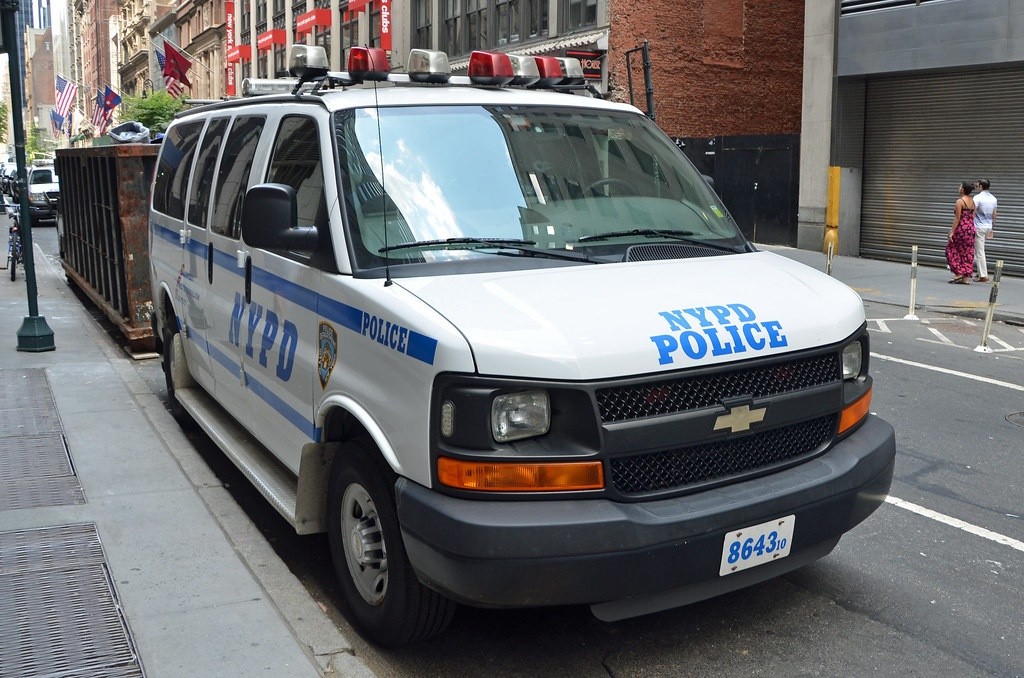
[
  {"xmin": 948, "ymin": 276, "xmax": 963, "ymax": 283},
  {"xmin": 955, "ymin": 281, "xmax": 970, "ymax": 285}
]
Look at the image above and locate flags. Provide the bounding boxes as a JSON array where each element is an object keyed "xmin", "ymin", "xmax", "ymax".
[
  {"xmin": 55, "ymin": 76, "xmax": 78, "ymax": 116},
  {"xmin": 50, "ymin": 110, "xmax": 63, "ymax": 138},
  {"xmin": 163, "ymin": 41, "xmax": 191, "ymax": 87},
  {"xmin": 155, "ymin": 50, "xmax": 184, "ymax": 99},
  {"xmin": 92, "ymin": 91, "xmax": 113, "ymax": 134},
  {"xmin": 104, "ymin": 86, "xmax": 122, "ymax": 121}
]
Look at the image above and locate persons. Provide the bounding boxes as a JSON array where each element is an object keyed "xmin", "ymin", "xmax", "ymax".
[
  {"xmin": 945, "ymin": 180, "xmax": 975, "ymax": 284},
  {"xmin": 973, "ymin": 178, "xmax": 997, "ymax": 281}
]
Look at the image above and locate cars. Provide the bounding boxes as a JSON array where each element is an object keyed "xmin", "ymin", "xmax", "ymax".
[{"xmin": 0, "ymin": 162, "xmax": 20, "ymax": 204}]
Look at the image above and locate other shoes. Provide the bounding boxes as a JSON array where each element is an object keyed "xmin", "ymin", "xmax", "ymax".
[{"xmin": 973, "ymin": 275, "xmax": 990, "ymax": 283}]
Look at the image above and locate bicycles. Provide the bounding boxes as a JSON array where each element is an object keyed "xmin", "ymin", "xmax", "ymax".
[{"xmin": 0, "ymin": 204, "xmax": 39, "ymax": 282}]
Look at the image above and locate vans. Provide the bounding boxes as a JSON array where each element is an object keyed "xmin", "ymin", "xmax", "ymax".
[
  {"xmin": 150, "ymin": 43, "xmax": 895, "ymax": 651},
  {"xmin": 27, "ymin": 159, "xmax": 62, "ymax": 226}
]
